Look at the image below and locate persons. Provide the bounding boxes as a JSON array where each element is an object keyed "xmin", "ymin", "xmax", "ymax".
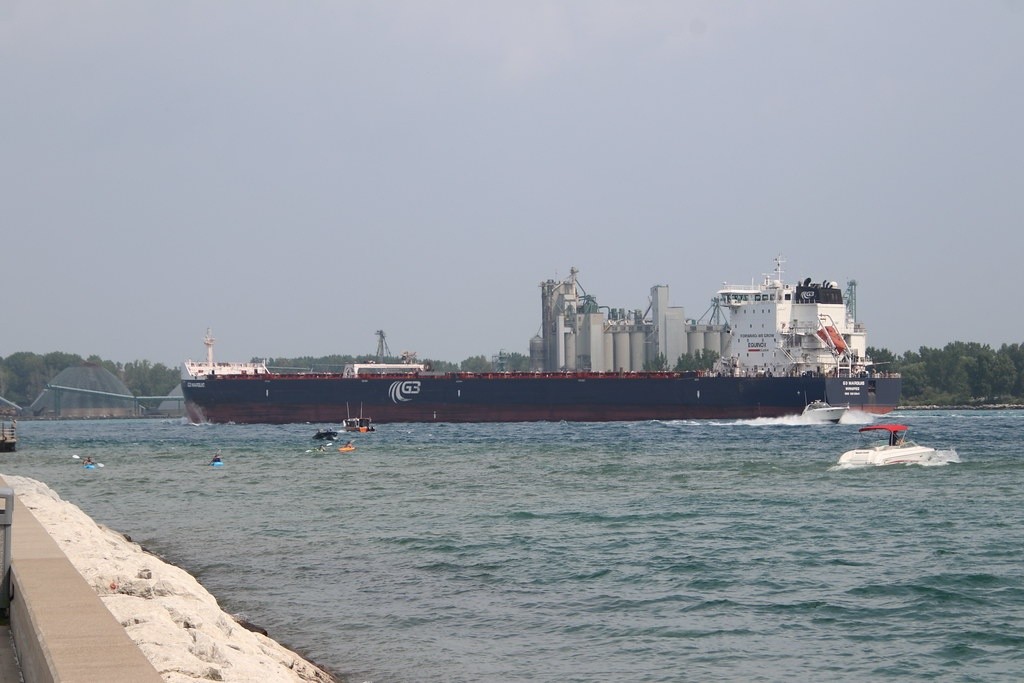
[
  {"xmin": 345, "ymin": 441, "xmax": 353, "ymax": 449},
  {"xmin": 83, "ymin": 457, "xmax": 96, "ymax": 466},
  {"xmin": 212, "ymin": 452, "xmax": 222, "ymax": 462},
  {"xmin": 889, "ymin": 430, "xmax": 900, "ymax": 446},
  {"xmin": 317, "ymin": 444, "xmax": 325, "ymax": 454},
  {"xmin": 10, "ymin": 418, "xmax": 17, "ymax": 440}
]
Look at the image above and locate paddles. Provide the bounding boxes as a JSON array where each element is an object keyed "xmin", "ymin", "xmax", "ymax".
[{"xmin": 72, "ymin": 454, "xmax": 105, "ymax": 468}]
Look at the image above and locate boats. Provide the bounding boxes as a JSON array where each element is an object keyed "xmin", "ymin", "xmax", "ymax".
[
  {"xmin": 84, "ymin": 462, "xmax": 104, "ymax": 470},
  {"xmin": 338, "ymin": 446, "xmax": 354, "ymax": 453},
  {"xmin": 312, "ymin": 428, "xmax": 338, "ymax": 438},
  {"xmin": 212, "ymin": 461, "xmax": 223, "ymax": 465},
  {"xmin": 800, "ymin": 390, "xmax": 847, "ymax": 424},
  {"xmin": 0, "ymin": 423, "xmax": 17, "ymax": 452},
  {"xmin": 835, "ymin": 424, "xmax": 938, "ymax": 465},
  {"xmin": 342, "ymin": 400, "xmax": 376, "ymax": 432}
]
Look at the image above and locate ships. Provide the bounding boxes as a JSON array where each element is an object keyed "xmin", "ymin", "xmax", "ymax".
[{"xmin": 177, "ymin": 254, "xmax": 904, "ymax": 423}]
